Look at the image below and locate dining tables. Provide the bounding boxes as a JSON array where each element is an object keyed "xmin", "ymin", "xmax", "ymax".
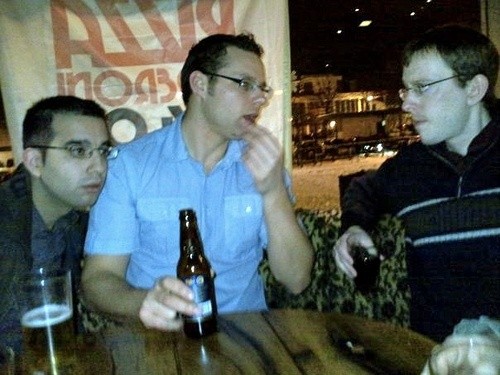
[{"xmin": 1, "ymin": 308, "xmax": 439, "ymax": 375}]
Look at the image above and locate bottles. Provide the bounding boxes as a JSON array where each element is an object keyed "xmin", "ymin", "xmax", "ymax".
[{"xmin": 177, "ymin": 208, "xmax": 220, "ymax": 340}]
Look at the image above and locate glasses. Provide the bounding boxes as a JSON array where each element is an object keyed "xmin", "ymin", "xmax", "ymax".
[
  {"xmin": 26, "ymin": 140, "xmax": 117, "ymax": 160},
  {"xmin": 396, "ymin": 74, "xmax": 474, "ymax": 101},
  {"xmin": 204, "ymin": 71, "xmax": 273, "ymax": 99}
]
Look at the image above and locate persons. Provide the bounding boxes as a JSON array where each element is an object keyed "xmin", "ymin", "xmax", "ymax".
[
  {"xmin": 419, "ymin": 318, "xmax": 500, "ymax": 375},
  {"xmin": 81, "ymin": 30, "xmax": 316, "ymax": 333},
  {"xmin": 329, "ymin": 22, "xmax": 499, "ymax": 343},
  {"xmin": 0, "ymin": 94, "xmax": 112, "ymax": 356}
]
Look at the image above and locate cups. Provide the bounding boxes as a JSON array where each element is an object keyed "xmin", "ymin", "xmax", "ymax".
[
  {"xmin": 12, "ymin": 266, "xmax": 79, "ymax": 374},
  {"xmin": 429, "ymin": 338, "xmax": 498, "ymax": 374}
]
